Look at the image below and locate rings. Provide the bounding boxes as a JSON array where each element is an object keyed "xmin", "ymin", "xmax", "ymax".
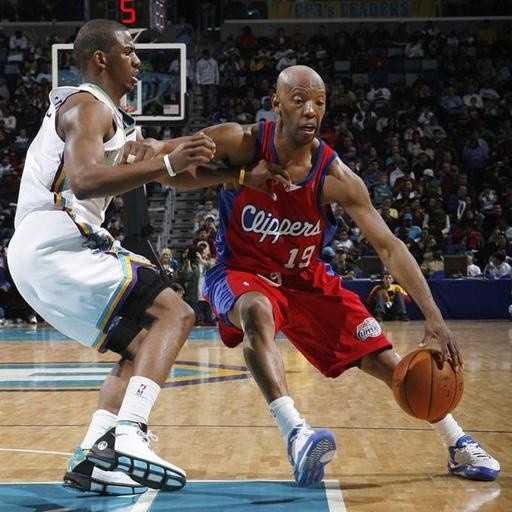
[{"xmin": 123, "ymin": 65, "xmax": 502, "ymax": 488}]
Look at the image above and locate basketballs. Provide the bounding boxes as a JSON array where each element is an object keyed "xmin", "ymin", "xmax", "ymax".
[{"xmin": 394, "ymin": 348, "xmax": 466, "ymax": 422}]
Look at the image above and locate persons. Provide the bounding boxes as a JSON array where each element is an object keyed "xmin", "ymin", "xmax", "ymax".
[
  {"xmin": 5, "ymin": 18, "xmax": 293, "ymax": 496},
  {"xmin": 1, "ymin": 27, "xmax": 83, "ymax": 326},
  {"xmin": 111, "ymin": 18, "xmax": 510, "ymax": 325}
]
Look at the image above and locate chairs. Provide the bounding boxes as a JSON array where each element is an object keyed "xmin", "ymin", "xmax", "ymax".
[{"xmin": 202, "ymin": 29, "xmax": 512, "ymax": 278}]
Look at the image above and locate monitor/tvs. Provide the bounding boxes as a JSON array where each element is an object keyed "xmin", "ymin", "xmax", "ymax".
[
  {"xmin": 444, "ymin": 255, "xmax": 467, "ymax": 277},
  {"xmin": 362, "ymin": 256, "xmax": 384, "ymax": 280}
]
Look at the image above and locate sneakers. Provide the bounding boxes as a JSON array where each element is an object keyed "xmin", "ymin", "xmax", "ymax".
[
  {"xmin": 62, "ymin": 423, "xmax": 186, "ymax": 496},
  {"xmin": 448, "ymin": 435, "xmax": 500, "ymax": 481},
  {"xmin": 288, "ymin": 421, "xmax": 336, "ymax": 487}
]
